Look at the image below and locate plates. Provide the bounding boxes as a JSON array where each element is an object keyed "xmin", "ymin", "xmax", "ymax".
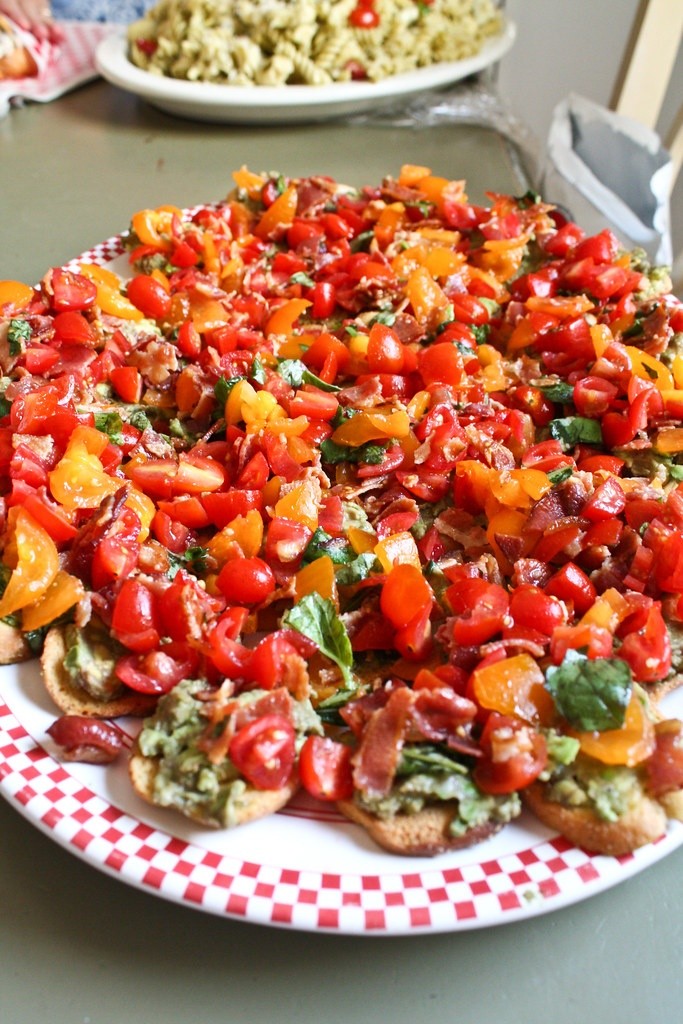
[
  {"xmin": 93, "ymin": 12, "xmax": 517, "ymax": 122},
  {"xmin": 0, "ymin": 202, "xmax": 683, "ymax": 935}
]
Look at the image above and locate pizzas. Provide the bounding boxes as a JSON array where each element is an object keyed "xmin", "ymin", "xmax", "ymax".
[{"xmin": 0, "ymin": 177, "xmax": 683, "ymax": 857}]
